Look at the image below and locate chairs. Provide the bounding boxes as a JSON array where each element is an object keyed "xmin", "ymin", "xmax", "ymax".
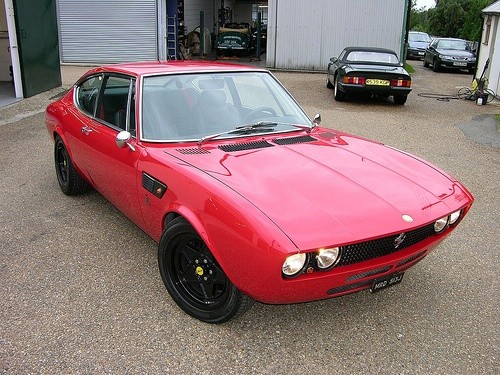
[
  {"xmin": 116, "ymin": 95, "xmax": 176, "ymax": 139},
  {"xmin": 192, "ymin": 90, "xmax": 240, "ymax": 136}
]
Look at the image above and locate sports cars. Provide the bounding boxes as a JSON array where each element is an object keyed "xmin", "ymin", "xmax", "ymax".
[{"xmin": 45, "ymin": 60, "xmax": 476, "ymax": 322}]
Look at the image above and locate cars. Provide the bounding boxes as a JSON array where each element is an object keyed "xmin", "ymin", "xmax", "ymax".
[
  {"xmin": 423, "ymin": 38, "xmax": 477, "ymax": 73},
  {"xmin": 326, "ymin": 46, "xmax": 412, "ymax": 106},
  {"xmin": 213, "ymin": 21, "xmax": 256, "ymax": 58},
  {"xmin": 404, "ymin": 31, "xmax": 434, "ymax": 59}
]
